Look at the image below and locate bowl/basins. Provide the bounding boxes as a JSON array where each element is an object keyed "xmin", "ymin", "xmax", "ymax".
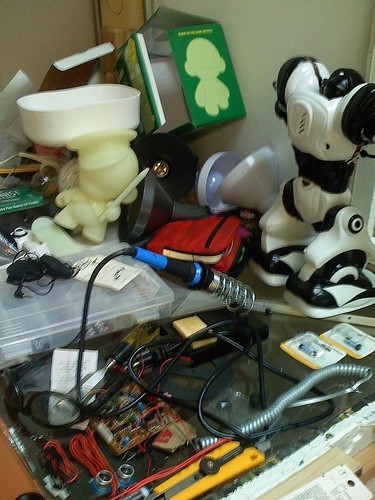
[{"xmin": 16, "ymin": 83, "xmax": 142, "ymax": 148}]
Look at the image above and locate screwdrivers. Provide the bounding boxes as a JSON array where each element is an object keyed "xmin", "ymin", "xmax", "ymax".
[{"xmin": 54, "ymin": 320, "xmax": 161, "ymax": 418}]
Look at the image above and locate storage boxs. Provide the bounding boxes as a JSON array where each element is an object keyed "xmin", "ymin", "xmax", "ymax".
[
  {"xmin": 0, "ymin": 242, "xmax": 175, "ymax": 367},
  {"xmin": 15, "ymin": 0, "xmax": 247, "ymax": 150}
]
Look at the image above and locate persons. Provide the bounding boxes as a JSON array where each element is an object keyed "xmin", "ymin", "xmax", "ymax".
[{"xmin": 248, "ymin": 55, "xmax": 375, "ymax": 319}]
